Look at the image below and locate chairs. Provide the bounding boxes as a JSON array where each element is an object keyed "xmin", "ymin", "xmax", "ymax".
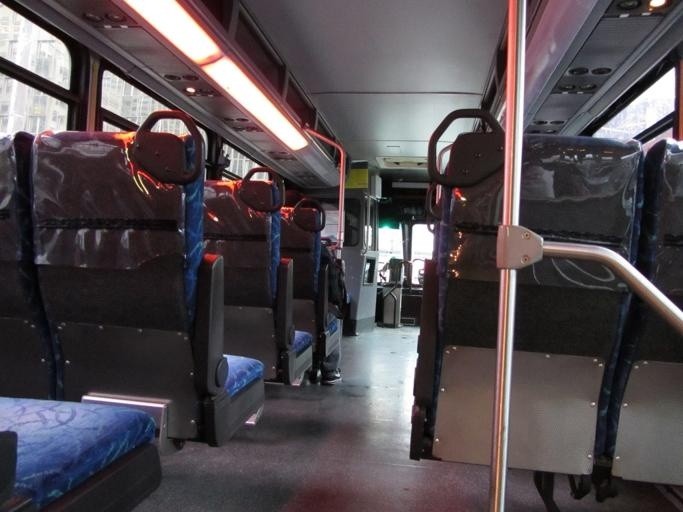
[
  {"xmin": 409, "ymin": 108, "xmax": 644, "ymax": 478},
  {"xmin": 27, "ymin": 111, "xmax": 266, "ymax": 446},
  {"xmin": 604, "ymin": 140, "xmax": 683, "ymax": 486},
  {"xmin": 281, "ymin": 199, "xmax": 342, "ymax": 357},
  {"xmin": 0, "ymin": 394, "xmax": 162, "ymax": 512},
  {"xmin": 204, "ymin": 166, "xmax": 314, "ymax": 387},
  {"xmin": 0, "ymin": 132, "xmax": 52, "ymax": 398}
]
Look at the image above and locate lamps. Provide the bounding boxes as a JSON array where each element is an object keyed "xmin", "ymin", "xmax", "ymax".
[
  {"xmin": 122, "ymin": 1, "xmax": 227, "ymax": 66},
  {"xmin": 199, "ymin": 55, "xmax": 309, "ymax": 152}
]
